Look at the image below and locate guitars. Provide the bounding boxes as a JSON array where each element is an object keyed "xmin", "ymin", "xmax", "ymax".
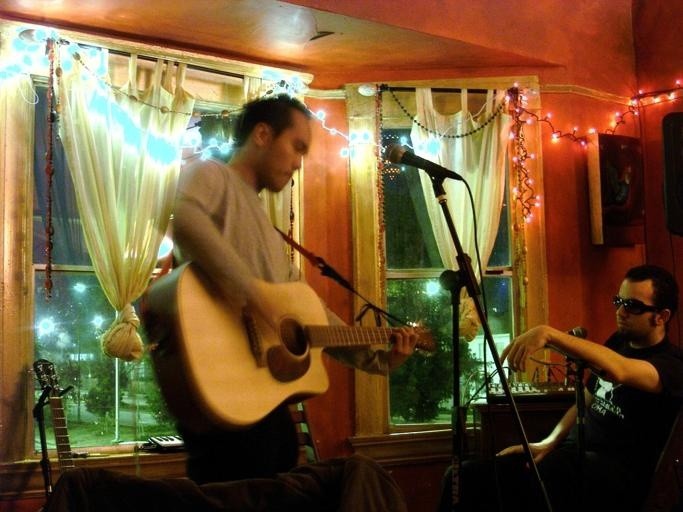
[
  {"xmin": 33, "ymin": 358, "xmax": 75, "ymax": 474},
  {"xmin": 138, "ymin": 258, "xmax": 440, "ymax": 437}
]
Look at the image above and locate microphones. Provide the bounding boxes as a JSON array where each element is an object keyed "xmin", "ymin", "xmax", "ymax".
[
  {"xmin": 384, "ymin": 143, "xmax": 464, "ymax": 179},
  {"xmin": 543, "ymin": 325, "xmax": 587, "ymax": 347}
]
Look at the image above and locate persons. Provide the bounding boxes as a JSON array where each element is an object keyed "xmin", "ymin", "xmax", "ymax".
[
  {"xmin": 484, "ymin": 268, "xmax": 673, "ymax": 499},
  {"xmin": 165, "ymin": 92, "xmax": 416, "ymax": 512}
]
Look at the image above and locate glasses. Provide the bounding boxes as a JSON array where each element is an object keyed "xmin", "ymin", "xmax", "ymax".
[{"xmin": 613, "ymin": 295, "xmax": 665, "ymax": 315}]
